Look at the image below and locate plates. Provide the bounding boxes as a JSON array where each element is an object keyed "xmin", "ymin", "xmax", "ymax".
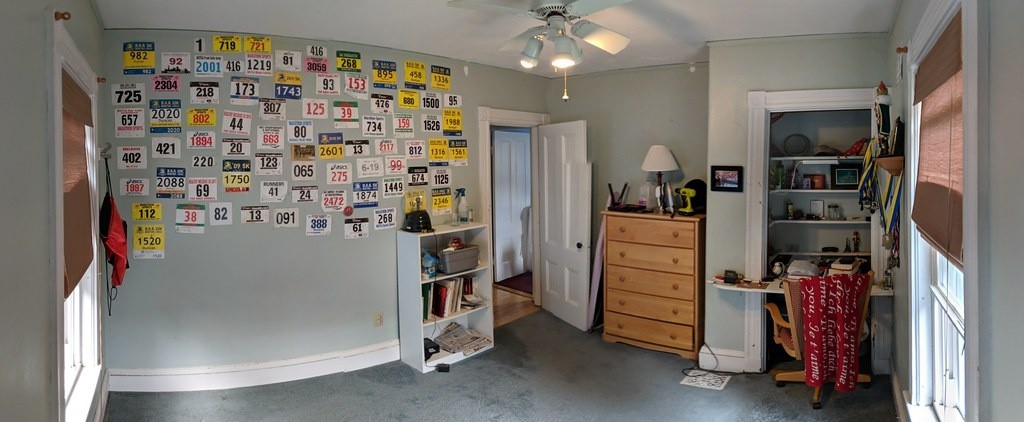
[{"xmin": 784, "ymin": 134, "xmax": 810, "ymax": 156}]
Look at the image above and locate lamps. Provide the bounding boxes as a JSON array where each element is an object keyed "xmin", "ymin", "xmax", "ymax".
[
  {"xmin": 519, "ymin": 33, "xmax": 584, "ymax": 69},
  {"xmin": 640, "ymin": 144, "xmax": 680, "ymax": 213}
]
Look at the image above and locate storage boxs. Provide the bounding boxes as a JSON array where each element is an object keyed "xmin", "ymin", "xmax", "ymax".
[{"xmin": 435, "ymin": 244, "xmax": 479, "ymax": 274}]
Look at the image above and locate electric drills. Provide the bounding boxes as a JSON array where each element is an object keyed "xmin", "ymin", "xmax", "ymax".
[{"xmin": 674, "ymin": 188, "xmax": 696, "ymax": 216}]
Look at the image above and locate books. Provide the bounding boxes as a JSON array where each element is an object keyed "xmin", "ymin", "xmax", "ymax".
[
  {"xmin": 422, "ymin": 273, "xmax": 482, "ymax": 321},
  {"xmin": 830, "ymin": 257, "xmax": 862, "ymax": 277}
]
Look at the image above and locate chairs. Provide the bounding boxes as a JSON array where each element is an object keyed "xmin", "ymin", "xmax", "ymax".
[{"xmin": 763, "ymin": 271, "xmax": 872, "ymax": 410}]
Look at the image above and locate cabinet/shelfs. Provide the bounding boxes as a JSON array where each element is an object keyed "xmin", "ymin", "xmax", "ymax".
[
  {"xmin": 397, "ymin": 221, "xmax": 494, "ymax": 374},
  {"xmin": 600, "ymin": 210, "xmax": 705, "ymax": 359},
  {"xmin": 769, "ymin": 156, "xmax": 871, "ymax": 257}
]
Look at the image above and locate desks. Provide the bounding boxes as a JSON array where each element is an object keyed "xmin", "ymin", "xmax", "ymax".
[{"xmin": 712, "ymin": 280, "xmax": 894, "ymax": 296}]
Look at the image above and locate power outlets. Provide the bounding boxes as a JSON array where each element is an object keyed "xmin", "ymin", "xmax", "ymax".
[{"xmin": 374, "ymin": 311, "xmax": 383, "ymax": 327}]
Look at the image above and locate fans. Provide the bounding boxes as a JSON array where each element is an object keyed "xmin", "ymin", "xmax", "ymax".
[{"xmin": 446, "ymin": 0, "xmax": 634, "ymax": 56}]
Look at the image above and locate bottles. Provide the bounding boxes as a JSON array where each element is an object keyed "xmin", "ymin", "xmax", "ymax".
[
  {"xmin": 786, "ymin": 200, "xmax": 793, "ymax": 220},
  {"xmin": 828, "ymin": 205, "xmax": 839, "ymax": 219},
  {"xmin": 638, "ymin": 180, "xmax": 656, "ymax": 212},
  {"xmin": 774, "ymin": 165, "xmax": 784, "ymax": 187}
]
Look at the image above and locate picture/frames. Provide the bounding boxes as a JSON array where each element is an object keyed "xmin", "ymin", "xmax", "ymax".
[
  {"xmin": 802, "ymin": 173, "xmax": 825, "ymax": 189},
  {"xmin": 830, "ymin": 162, "xmax": 862, "ymax": 190},
  {"xmin": 710, "ymin": 165, "xmax": 743, "ymax": 193}
]
